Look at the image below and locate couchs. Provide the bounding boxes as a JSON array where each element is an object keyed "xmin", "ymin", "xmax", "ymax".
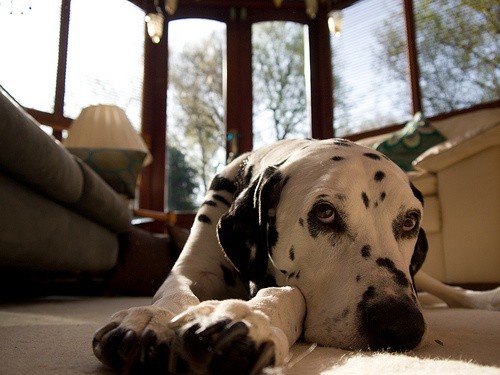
[
  {"xmin": 343, "ymin": 99, "xmax": 500, "ymax": 285},
  {"xmin": 0, "ymin": 86, "xmax": 156, "ymax": 290}
]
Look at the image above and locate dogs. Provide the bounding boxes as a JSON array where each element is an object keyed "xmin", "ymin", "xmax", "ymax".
[{"xmin": 91, "ymin": 137, "xmax": 500, "ymax": 375}]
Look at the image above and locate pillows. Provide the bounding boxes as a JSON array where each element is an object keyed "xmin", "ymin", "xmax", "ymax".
[
  {"xmin": 66, "ymin": 148, "xmax": 147, "ymax": 199},
  {"xmin": 410, "ymin": 118, "xmax": 499, "ymax": 174},
  {"xmin": 167, "ymin": 224, "xmax": 191, "ymax": 255},
  {"xmin": 374, "ymin": 111, "xmax": 448, "ymax": 174}
]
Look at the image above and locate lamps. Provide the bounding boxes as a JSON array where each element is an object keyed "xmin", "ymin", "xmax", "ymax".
[
  {"xmin": 145, "ymin": 0, "xmax": 165, "ymax": 43},
  {"xmin": 64, "ymin": 105, "xmax": 153, "ymax": 167},
  {"xmin": 326, "ymin": 0, "xmax": 343, "ymax": 37}
]
